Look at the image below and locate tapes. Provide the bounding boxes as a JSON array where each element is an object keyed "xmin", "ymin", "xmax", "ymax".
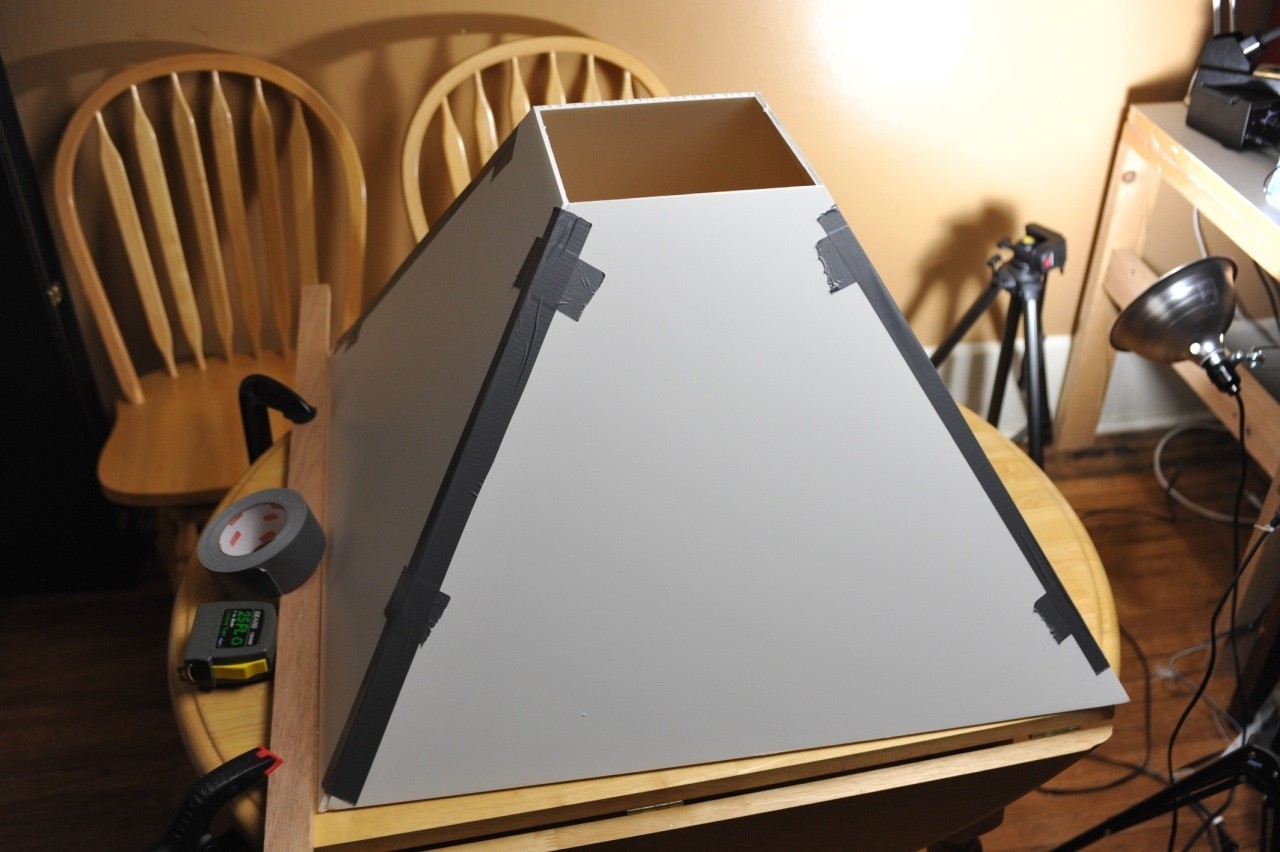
[{"xmin": 198, "ymin": 488, "xmax": 326, "ymax": 601}]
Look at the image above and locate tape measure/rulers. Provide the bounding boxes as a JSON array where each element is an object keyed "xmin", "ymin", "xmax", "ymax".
[{"xmin": 175, "ymin": 597, "xmax": 277, "ymax": 693}]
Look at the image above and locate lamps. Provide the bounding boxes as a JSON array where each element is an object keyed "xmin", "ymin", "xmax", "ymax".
[{"xmin": 1111, "ymin": 256, "xmax": 1241, "ymax": 403}]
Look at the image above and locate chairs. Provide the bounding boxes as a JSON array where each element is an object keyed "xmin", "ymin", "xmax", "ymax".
[
  {"xmin": 400, "ymin": 37, "xmax": 669, "ymax": 246},
  {"xmin": 53, "ymin": 49, "xmax": 370, "ymax": 586}
]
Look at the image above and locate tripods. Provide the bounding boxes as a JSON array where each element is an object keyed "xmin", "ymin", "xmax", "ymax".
[{"xmin": 929, "ymin": 248, "xmax": 1051, "ymax": 469}]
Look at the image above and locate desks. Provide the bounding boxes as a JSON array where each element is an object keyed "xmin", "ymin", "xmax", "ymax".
[
  {"xmin": 169, "ymin": 384, "xmax": 1120, "ymax": 851},
  {"xmin": 1058, "ymin": 101, "xmax": 1279, "ymax": 684}
]
